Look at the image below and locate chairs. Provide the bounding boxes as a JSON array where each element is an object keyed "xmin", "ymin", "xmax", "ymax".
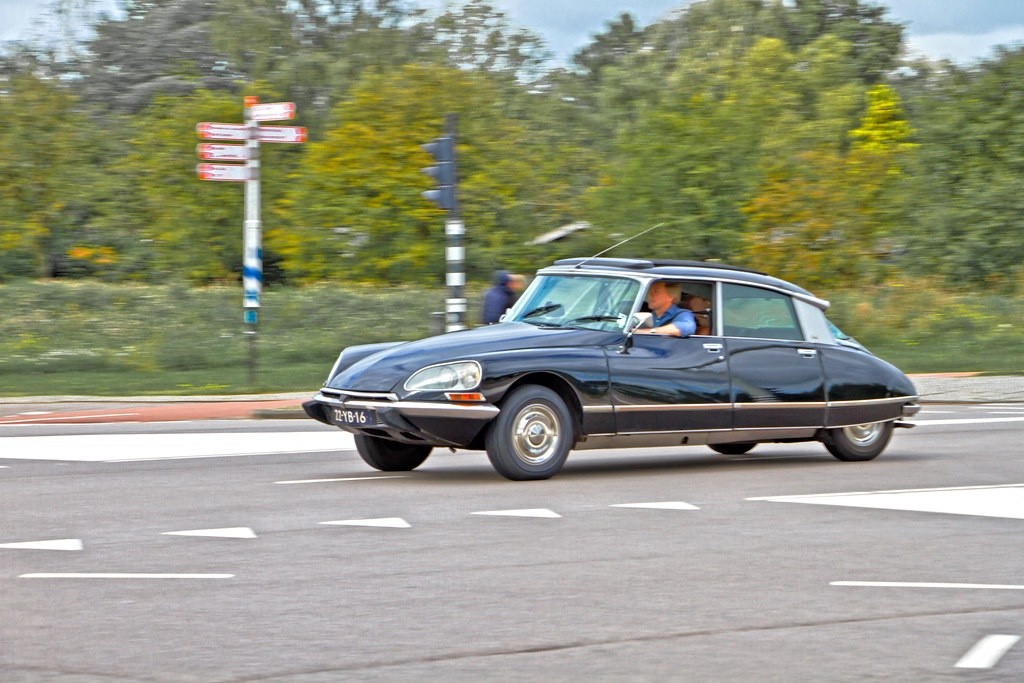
[
  {"xmin": 679, "ymin": 295, "xmax": 711, "ymax": 336},
  {"xmin": 617, "ymin": 301, "xmax": 648, "ymax": 315}
]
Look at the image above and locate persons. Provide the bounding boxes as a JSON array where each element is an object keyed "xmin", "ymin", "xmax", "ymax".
[
  {"xmin": 483, "ymin": 271, "xmax": 517, "ymax": 325},
  {"xmin": 633, "ymin": 281, "xmax": 696, "ymax": 339}
]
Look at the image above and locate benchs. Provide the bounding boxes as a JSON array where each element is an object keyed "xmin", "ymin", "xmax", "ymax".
[{"xmin": 724, "ymin": 324, "xmax": 799, "ymax": 340}]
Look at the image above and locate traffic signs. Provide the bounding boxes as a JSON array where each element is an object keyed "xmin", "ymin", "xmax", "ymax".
[{"xmin": 192, "ymin": 91, "xmax": 310, "ymax": 180}]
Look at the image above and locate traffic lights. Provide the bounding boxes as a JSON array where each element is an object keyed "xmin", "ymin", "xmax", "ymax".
[{"xmin": 422, "ymin": 136, "xmax": 454, "ymax": 210}]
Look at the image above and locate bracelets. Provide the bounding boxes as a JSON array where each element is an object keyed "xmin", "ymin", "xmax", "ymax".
[{"xmin": 650, "ymin": 327, "xmax": 655, "ymax": 333}]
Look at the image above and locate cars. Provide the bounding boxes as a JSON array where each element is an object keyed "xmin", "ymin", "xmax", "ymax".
[{"xmin": 300, "ymin": 256, "xmax": 920, "ymax": 482}]
